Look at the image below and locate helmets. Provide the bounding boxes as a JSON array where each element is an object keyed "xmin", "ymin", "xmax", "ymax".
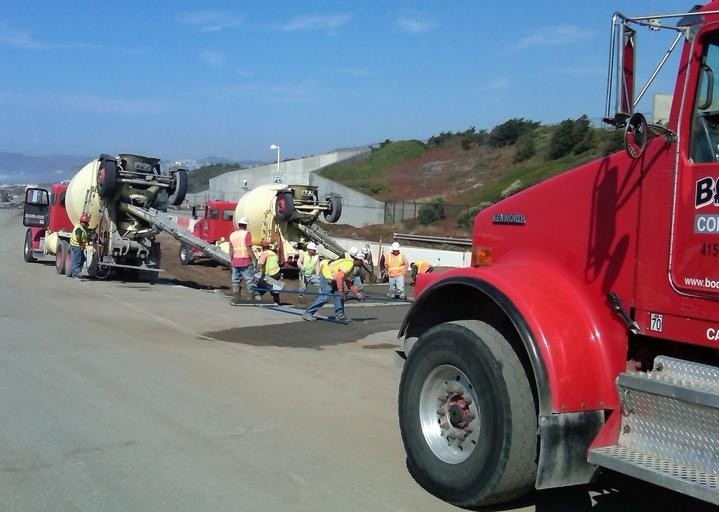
[
  {"xmin": 79, "ymin": 215, "xmax": 89, "ymax": 222},
  {"xmin": 259, "ymin": 242, "xmax": 270, "ymax": 247},
  {"xmin": 391, "ymin": 242, "xmax": 400, "ymax": 250},
  {"xmin": 349, "ymin": 247, "xmax": 368, "ymax": 260},
  {"xmin": 306, "ymin": 241, "xmax": 318, "ymax": 250},
  {"xmin": 238, "ymin": 217, "xmax": 249, "ymax": 224}
]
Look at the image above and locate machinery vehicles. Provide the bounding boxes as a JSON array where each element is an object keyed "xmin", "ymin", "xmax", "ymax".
[
  {"xmin": 175, "ymin": 176, "xmax": 374, "ymax": 279},
  {"xmin": 22, "ymin": 151, "xmax": 289, "ymax": 297},
  {"xmin": 397, "ymin": 2, "xmax": 718, "ymax": 508}
]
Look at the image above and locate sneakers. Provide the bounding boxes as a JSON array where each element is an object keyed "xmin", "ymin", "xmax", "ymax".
[
  {"xmin": 335, "ymin": 315, "xmax": 351, "ymax": 323},
  {"xmin": 303, "ymin": 312, "xmax": 317, "ymax": 322}
]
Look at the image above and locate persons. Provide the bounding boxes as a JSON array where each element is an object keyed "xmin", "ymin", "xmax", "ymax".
[
  {"xmin": 229, "ymin": 217, "xmax": 258, "ymax": 296},
  {"xmin": 252, "ymin": 242, "xmax": 281, "ymax": 305},
  {"xmin": 384, "ymin": 242, "xmax": 409, "ymax": 300},
  {"xmin": 296, "ymin": 241, "xmax": 367, "ymax": 322},
  {"xmin": 67, "ymin": 215, "xmax": 90, "ymax": 278},
  {"xmin": 410, "ymin": 262, "xmax": 433, "ymax": 283}
]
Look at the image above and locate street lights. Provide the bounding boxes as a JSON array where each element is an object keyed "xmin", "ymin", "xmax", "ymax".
[{"xmin": 268, "ymin": 144, "xmax": 282, "ymax": 173}]
